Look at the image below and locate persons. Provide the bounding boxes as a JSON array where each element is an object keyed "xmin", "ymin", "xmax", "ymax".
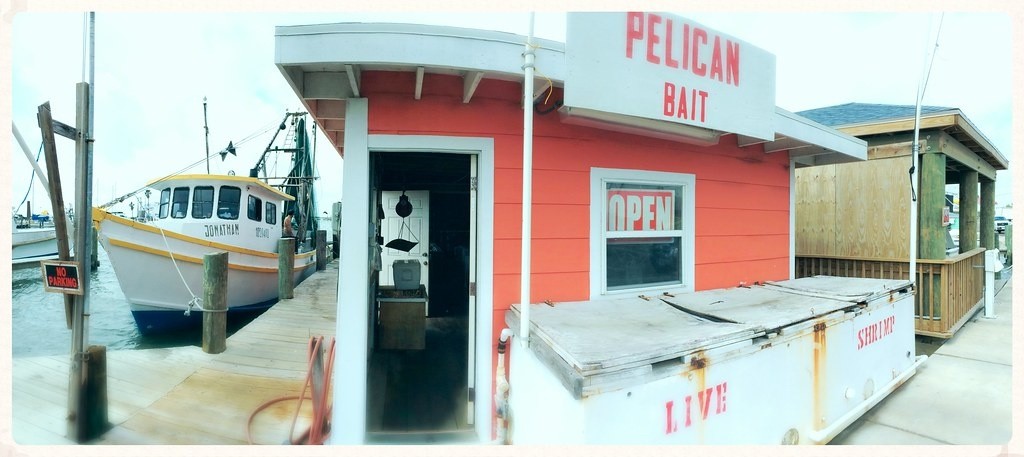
[{"xmin": 283, "ymin": 210, "xmax": 294, "ymax": 237}]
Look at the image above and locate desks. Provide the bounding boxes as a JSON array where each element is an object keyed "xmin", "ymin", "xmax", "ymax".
[{"xmin": 376, "ymin": 284, "xmax": 428, "ymax": 350}]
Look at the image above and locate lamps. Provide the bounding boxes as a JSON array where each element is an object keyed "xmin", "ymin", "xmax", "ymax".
[{"xmin": 557, "ymin": 105, "xmax": 721, "ymax": 146}]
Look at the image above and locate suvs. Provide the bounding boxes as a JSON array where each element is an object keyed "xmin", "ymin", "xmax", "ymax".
[{"xmin": 994, "ymin": 216, "xmax": 1009, "ymax": 231}]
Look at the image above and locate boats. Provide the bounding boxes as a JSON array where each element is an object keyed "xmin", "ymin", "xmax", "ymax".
[
  {"xmin": 92, "ymin": 95, "xmax": 335, "ymax": 339},
  {"xmin": 12, "ymin": 213, "xmax": 78, "ymax": 265}
]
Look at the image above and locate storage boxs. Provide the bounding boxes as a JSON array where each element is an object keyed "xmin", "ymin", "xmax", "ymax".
[{"xmin": 393, "ymin": 259, "xmax": 421, "ymax": 290}]
